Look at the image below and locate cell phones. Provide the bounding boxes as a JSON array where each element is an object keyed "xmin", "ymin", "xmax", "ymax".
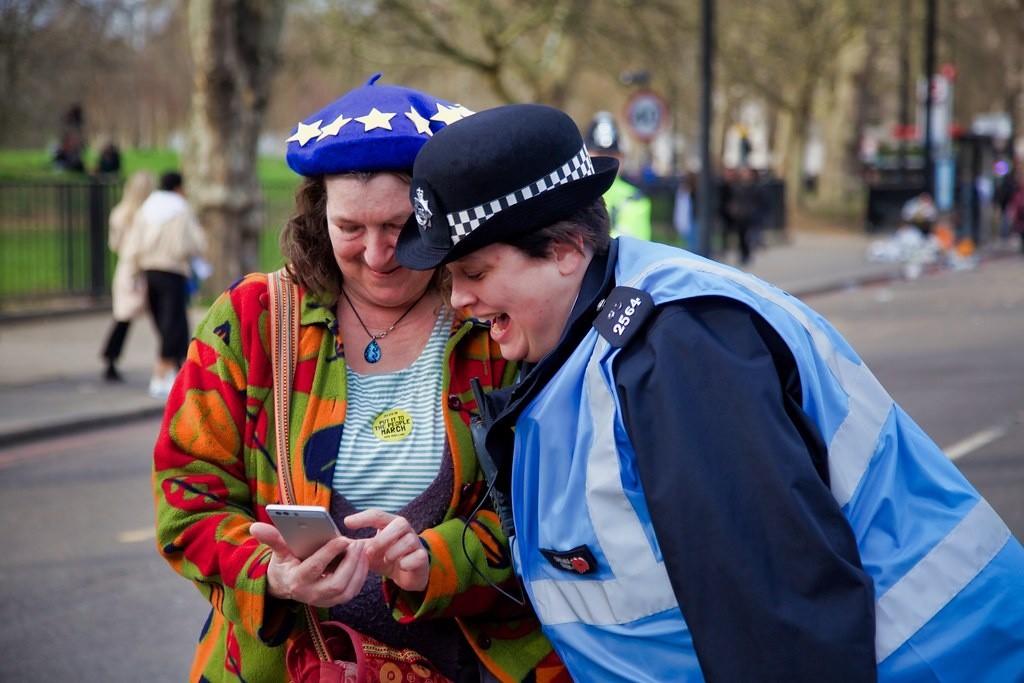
[{"xmin": 266, "ymin": 505, "xmax": 347, "ymax": 564}]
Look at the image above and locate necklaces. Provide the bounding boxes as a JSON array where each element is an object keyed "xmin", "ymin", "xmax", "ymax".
[{"xmin": 343, "ymin": 289, "xmax": 429, "ymax": 364}]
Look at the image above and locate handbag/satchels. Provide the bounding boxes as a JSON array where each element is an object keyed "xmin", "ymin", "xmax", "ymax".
[{"xmin": 285, "ymin": 621, "xmax": 450, "ymax": 683}]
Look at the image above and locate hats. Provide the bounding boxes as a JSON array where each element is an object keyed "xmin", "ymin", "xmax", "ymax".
[
  {"xmin": 287, "ymin": 71, "xmax": 482, "ymax": 177},
  {"xmin": 396, "ymin": 103, "xmax": 621, "ymax": 273}
]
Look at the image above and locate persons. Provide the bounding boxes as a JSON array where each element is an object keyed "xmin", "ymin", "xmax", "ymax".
[
  {"xmin": 583, "ymin": 120, "xmax": 652, "ymax": 242},
  {"xmin": 670, "ymin": 167, "xmax": 762, "ymax": 264},
  {"xmin": 1004, "ymin": 136, "xmax": 1024, "ymax": 240},
  {"xmin": 119, "ymin": 173, "xmax": 208, "ymax": 394},
  {"xmin": 102, "ymin": 173, "xmax": 155, "ymax": 382},
  {"xmin": 151, "ymin": 75, "xmax": 579, "ymax": 682},
  {"xmin": 397, "ymin": 103, "xmax": 1024, "ymax": 683},
  {"xmin": 51, "ymin": 125, "xmax": 91, "ymax": 172},
  {"xmin": 900, "ymin": 192, "xmax": 938, "ymax": 233}
]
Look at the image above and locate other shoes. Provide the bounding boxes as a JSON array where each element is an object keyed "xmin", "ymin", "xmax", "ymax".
[{"xmin": 147, "ymin": 369, "xmax": 178, "ymax": 396}]
